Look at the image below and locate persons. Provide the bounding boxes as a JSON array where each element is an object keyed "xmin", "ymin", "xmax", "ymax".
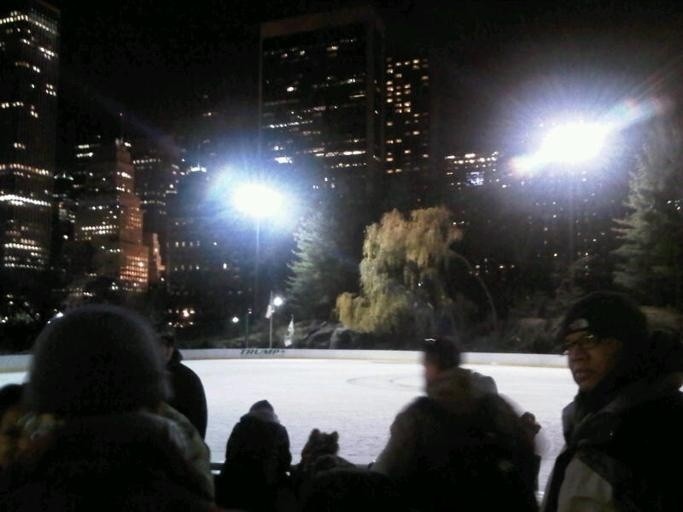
[
  {"xmin": 142, "ymin": 324, "xmax": 208, "ymax": 438},
  {"xmin": 0, "ymin": 301, "xmax": 221, "ymax": 510},
  {"xmin": 220, "ymin": 397, "xmax": 292, "ymax": 511},
  {"xmin": 286, "ymin": 426, "xmax": 353, "ymax": 511},
  {"xmin": 364, "ymin": 335, "xmax": 544, "ymax": 509},
  {"xmin": 531, "ymin": 287, "xmax": 681, "ymax": 509}
]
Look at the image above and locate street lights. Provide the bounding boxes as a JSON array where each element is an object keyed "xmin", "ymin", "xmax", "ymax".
[
  {"xmin": 551, "ymin": 111, "xmax": 578, "ymax": 278},
  {"xmin": 235, "ymin": 174, "xmax": 270, "ymax": 346}
]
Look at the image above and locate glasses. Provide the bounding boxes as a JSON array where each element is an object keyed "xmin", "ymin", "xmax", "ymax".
[{"xmin": 559, "ymin": 329, "xmax": 600, "ymax": 356}]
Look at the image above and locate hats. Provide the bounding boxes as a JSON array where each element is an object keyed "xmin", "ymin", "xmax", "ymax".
[
  {"xmin": 22, "ymin": 301, "xmax": 168, "ymax": 415},
  {"xmin": 561, "ymin": 292, "xmax": 648, "ymax": 346}
]
